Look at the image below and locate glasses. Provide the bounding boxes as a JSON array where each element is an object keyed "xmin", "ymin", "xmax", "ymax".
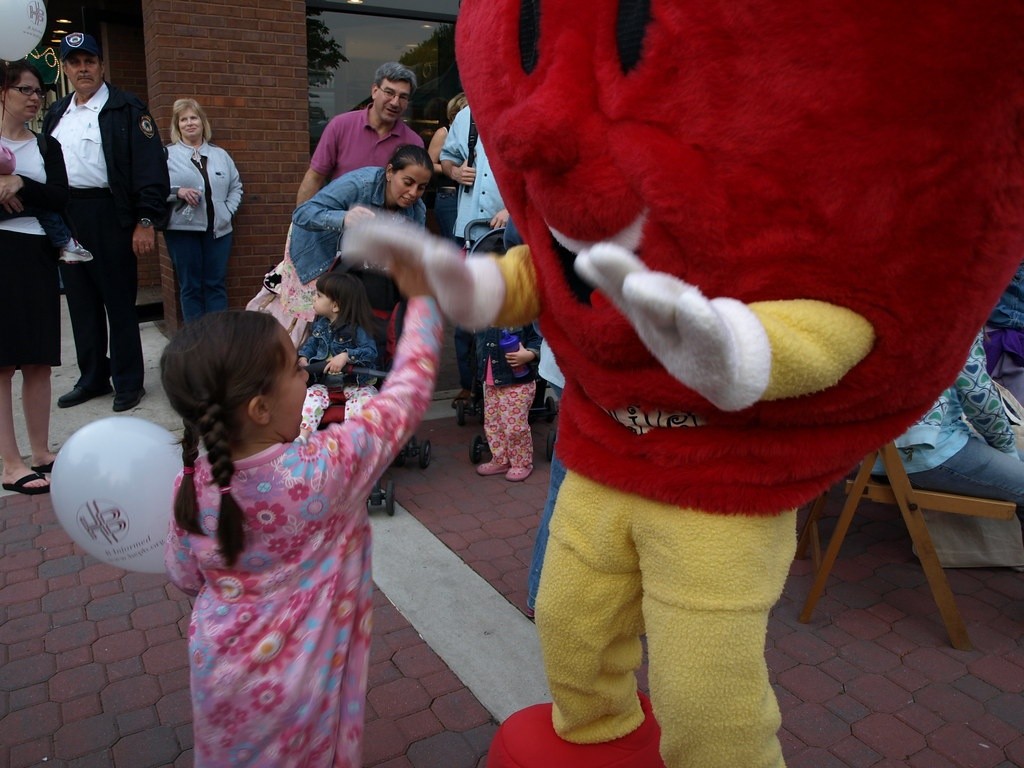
[
  {"xmin": 377, "ymin": 84, "xmax": 411, "ymax": 104},
  {"xmin": 1, "ymin": 86, "xmax": 42, "ymax": 98}
]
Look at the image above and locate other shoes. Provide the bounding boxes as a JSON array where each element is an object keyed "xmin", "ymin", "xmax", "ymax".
[
  {"xmin": 505, "ymin": 464, "xmax": 533, "ymax": 481},
  {"xmin": 477, "ymin": 458, "xmax": 508, "ymax": 475}
]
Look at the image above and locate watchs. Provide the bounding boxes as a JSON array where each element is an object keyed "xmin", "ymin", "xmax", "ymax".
[{"xmin": 139, "ymin": 218, "xmax": 153, "ymax": 227}]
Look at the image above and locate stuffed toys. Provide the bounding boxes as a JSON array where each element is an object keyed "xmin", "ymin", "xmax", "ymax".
[{"xmin": 341, "ymin": 1, "xmax": 1024, "ymax": 768}]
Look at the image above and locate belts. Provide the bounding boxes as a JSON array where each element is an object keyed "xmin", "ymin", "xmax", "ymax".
[
  {"xmin": 437, "ymin": 188, "xmax": 455, "ymax": 193},
  {"xmin": 68, "ymin": 188, "xmax": 111, "ymax": 198}
]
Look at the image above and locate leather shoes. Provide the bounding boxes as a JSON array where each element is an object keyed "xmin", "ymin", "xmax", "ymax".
[
  {"xmin": 113, "ymin": 387, "xmax": 145, "ymax": 412},
  {"xmin": 58, "ymin": 383, "xmax": 113, "ymax": 408}
]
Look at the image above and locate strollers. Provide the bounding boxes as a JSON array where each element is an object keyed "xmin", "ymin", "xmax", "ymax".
[
  {"xmin": 454, "ymin": 218, "xmax": 558, "ymax": 465},
  {"xmin": 296, "ymin": 230, "xmax": 432, "ymax": 517}
]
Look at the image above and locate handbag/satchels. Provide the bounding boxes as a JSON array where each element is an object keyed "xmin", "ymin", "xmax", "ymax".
[
  {"xmin": 245, "ymin": 261, "xmax": 311, "ymax": 351},
  {"xmin": 150, "ymin": 147, "xmax": 170, "ymax": 230}
]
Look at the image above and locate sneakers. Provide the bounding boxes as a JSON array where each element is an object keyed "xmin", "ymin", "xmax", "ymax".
[{"xmin": 58, "ymin": 240, "xmax": 93, "ymax": 264}]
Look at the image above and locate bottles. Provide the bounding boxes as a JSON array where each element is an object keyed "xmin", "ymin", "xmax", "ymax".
[
  {"xmin": 181, "ymin": 186, "xmax": 204, "ymax": 222},
  {"xmin": 499, "ymin": 329, "xmax": 529, "ymax": 378},
  {"xmin": 325, "ymin": 372, "xmax": 345, "ymax": 393}
]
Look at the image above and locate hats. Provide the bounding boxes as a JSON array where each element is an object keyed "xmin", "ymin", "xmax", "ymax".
[{"xmin": 60, "ymin": 32, "xmax": 101, "ymax": 61}]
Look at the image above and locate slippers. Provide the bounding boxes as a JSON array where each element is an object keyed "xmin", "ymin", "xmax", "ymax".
[
  {"xmin": 3, "ymin": 473, "xmax": 49, "ymax": 494},
  {"xmin": 452, "ymin": 395, "xmax": 471, "ymax": 408},
  {"xmin": 30, "ymin": 461, "xmax": 54, "ymax": 472}
]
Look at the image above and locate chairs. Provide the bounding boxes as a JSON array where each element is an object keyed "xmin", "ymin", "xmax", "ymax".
[{"xmin": 795, "ymin": 437, "xmax": 1017, "ymax": 652}]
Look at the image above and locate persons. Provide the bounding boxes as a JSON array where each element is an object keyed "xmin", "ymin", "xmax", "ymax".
[
  {"xmin": 160, "ymin": 249, "xmax": 443, "ymax": 768},
  {"xmin": 41, "ymin": 32, "xmax": 170, "ymax": 412},
  {"xmin": 427, "ymin": 94, "xmax": 565, "ymax": 613},
  {"xmin": 861, "ymin": 261, "xmax": 1024, "ymax": 507},
  {"xmin": 297, "ymin": 275, "xmax": 378, "ymax": 442},
  {"xmin": 285, "ymin": 143, "xmax": 433, "ymax": 318},
  {"xmin": 296, "ymin": 63, "xmax": 424, "ymax": 209},
  {"xmin": 0, "ymin": 60, "xmax": 71, "ymax": 495},
  {"xmin": 163, "ymin": 99, "xmax": 244, "ymax": 325}
]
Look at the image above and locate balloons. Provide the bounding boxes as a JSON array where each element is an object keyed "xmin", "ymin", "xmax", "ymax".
[{"xmin": 0, "ymin": 0, "xmax": 47, "ymax": 60}]
